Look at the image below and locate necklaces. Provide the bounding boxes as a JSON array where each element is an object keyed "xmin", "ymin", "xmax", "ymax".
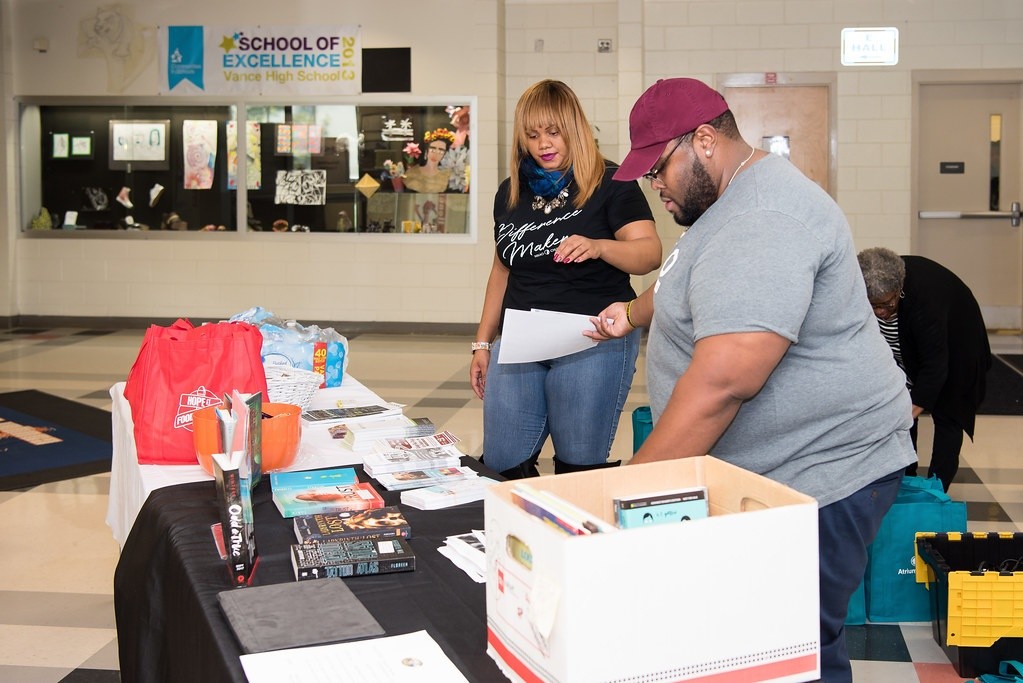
[
  {"xmin": 729, "ymin": 146, "xmax": 754, "ymax": 184},
  {"xmin": 530, "ymin": 180, "xmax": 572, "ymax": 214}
]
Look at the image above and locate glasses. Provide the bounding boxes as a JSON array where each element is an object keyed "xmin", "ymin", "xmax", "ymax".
[
  {"xmin": 643, "ymin": 134, "xmax": 688, "ymax": 180},
  {"xmin": 871, "ymin": 293, "xmax": 899, "ymax": 310}
]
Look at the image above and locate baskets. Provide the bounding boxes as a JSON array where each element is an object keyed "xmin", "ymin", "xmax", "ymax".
[{"xmin": 262, "ymin": 366, "xmax": 325, "ymax": 413}]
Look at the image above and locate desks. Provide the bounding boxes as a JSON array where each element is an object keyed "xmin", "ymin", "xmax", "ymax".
[{"xmin": 103, "ymin": 374, "xmax": 853, "ymax": 683}]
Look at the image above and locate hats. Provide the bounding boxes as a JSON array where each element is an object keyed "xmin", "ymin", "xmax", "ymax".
[{"xmin": 612, "ymin": 78, "xmax": 729, "ymax": 182}]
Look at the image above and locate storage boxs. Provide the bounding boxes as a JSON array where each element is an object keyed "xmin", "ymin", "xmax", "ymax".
[
  {"xmin": 912, "ymin": 531, "xmax": 1023, "ymax": 679},
  {"xmin": 866, "ymin": 473, "xmax": 967, "ymax": 623},
  {"xmin": 483, "ymin": 454, "xmax": 820, "ymax": 683},
  {"xmin": 841, "ymin": 577, "xmax": 867, "ymax": 625}
]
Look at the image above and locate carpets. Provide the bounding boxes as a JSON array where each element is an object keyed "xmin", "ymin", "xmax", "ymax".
[{"xmin": 0, "ymin": 388, "xmax": 112, "ymax": 492}]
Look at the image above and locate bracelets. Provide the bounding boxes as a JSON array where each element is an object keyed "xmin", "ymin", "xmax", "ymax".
[{"xmin": 626, "ymin": 300, "xmax": 638, "ymax": 329}]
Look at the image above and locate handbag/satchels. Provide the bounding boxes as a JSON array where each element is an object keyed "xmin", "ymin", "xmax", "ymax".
[
  {"xmin": 123, "ymin": 319, "xmax": 271, "ymax": 467},
  {"xmin": 844, "ymin": 475, "xmax": 968, "ymax": 626}
]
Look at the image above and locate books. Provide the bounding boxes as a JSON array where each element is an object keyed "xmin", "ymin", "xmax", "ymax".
[
  {"xmin": 270, "ymin": 468, "xmax": 416, "ymax": 581},
  {"xmin": 437, "ymin": 529, "xmax": 486, "ymax": 582},
  {"xmin": 211, "ymin": 389, "xmax": 263, "ymax": 586},
  {"xmin": 613, "ymin": 486, "xmax": 709, "ymax": 529},
  {"xmin": 511, "ymin": 483, "xmax": 618, "ymax": 537}
]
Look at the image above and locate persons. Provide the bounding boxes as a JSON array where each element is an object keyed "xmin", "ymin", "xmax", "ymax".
[
  {"xmin": 859, "ymin": 247, "xmax": 992, "ymax": 493},
  {"xmin": 470, "ymin": 80, "xmax": 662, "ymax": 478},
  {"xmin": 583, "ymin": 77, "xmax": 918, "ymax": 683}
]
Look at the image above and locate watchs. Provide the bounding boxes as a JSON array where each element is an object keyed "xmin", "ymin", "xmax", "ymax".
[{"xmin": 471, "ymin": 342, "xmax": 492, "ymax": 354}]
